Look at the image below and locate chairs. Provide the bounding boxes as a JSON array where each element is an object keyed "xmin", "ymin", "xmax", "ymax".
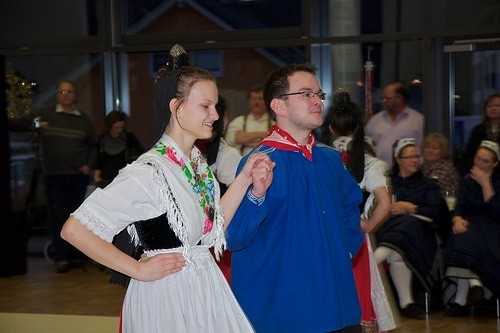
[{"xmin": 442, "ymin": 185, "xmax": 482, "ymax": 308}]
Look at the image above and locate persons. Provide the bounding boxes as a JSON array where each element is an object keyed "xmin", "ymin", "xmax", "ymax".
[
  {"xmin": 61, "ymin": 61, "xmax": 260, "ymax": 332},
  {"xmin": 199, "ymin": 87, "xmax": 275, "ymax": 196},
  {"xmin": 219, "ymin": 62, "xmax": 364, "ymax": 333},
  {"xmin": 36, "ymin": 79, "xmax": 94, "ymax": 272},
  {"xmin": 94, "ymin": 110, "xmax": 148, "ymax": 270},
  {"xmin": 5, "ymin": 71, "xmax": 48, "ymax": 275},
  {"xmin": 320, "ymin": 93, "xmax": 397, "ymax": 333},
  {"xmin": 369, "ymin": 136, "xmax": 450, "ymax": 322},
  {"xmin": 444, "ymin": 137, "xmax": 500, "ymax": 319},
  {"xmin": 366, "ymin": 80, "xmax": 500, "ymax": 204}
]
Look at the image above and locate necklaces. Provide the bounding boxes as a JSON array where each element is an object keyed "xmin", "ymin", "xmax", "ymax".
[{"xmin": 100, "ymin": 135, "xmax": 118, "ymax": 153}]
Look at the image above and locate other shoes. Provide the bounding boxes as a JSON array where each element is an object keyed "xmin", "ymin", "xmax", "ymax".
[
  {"xmin": 474, "ymin": 296, "xmax": 497, "ymax": 318},
  {"xmin": 55, "ymin": 260, "xmax": 86, "ymax": 272},
  {"xmin": 443, "ymin": 302, "xmax": 472, "ymax": 318},
  {"xmin": 401, "ymin": 302, "xmax": 427, "ymax": 319}
]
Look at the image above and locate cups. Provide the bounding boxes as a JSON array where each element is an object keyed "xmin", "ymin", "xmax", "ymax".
[{"xmin": 443, "ymin": 184, "xmax": 458, "ymax": 211}]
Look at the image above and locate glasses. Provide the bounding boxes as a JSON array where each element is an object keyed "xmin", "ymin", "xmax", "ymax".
[
  {"xmin": 56, "ymin": 90, "xmax": 76, "ymax": 95},
  {"xmin": 276, "ymin": 90, "xmax": 327, "ymax": 100},
  {"xmin": 398, "ymin": 155, "xmax": 420, "ymax": 159}
]
